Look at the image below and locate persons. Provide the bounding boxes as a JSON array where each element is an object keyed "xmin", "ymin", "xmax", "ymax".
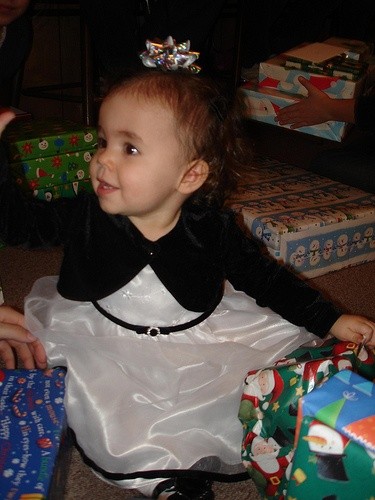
[
  {"xmin": 0, "ymin": 1, "xmax": 52, "ymax": 243},
  {"xmin": 46, "ymin": 68, "xmax": 375, "ymax": 500},
  {"xmin": 274, "ymin": 76, "xmax": 375, "ymax": 130},
  {"xmin": 0, "ymin": 108, "xmax": 50, "ymax": 500}
]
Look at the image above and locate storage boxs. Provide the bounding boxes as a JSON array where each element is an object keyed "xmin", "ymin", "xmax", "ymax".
[
  {"xmin": 0, "ymin": 366, "xmax": 67, "ymax": 500},
  {"xmin": 222, "ymin": 157, "xmax": 375, "ymax": 279},
  {"xmin": 4, "ymin": 118, "xmax": 99, "ymax": 201},
  {"xmin": 236, "ymin": 78, "xmax": 375, "ymax": 143},
  {"xmin": 258, "ymin": 41, "xmax": 375, "ymax": 99}
]
